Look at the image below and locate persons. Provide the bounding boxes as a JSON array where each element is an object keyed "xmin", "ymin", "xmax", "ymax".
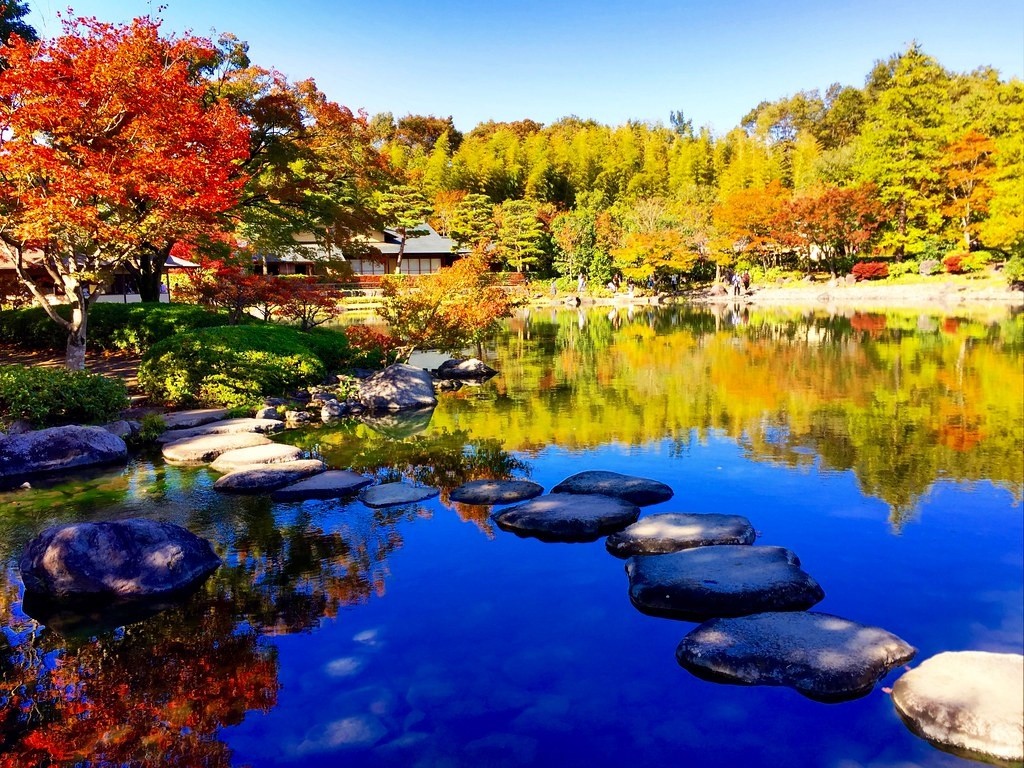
[
  {"xmin": 80, "ymin": 279, "xmax": 182, "ymax": 303},
  {"xmin": 732, "ymin": 271, "xmax": 742, "ymax": 295},
  {"xmin": 512, "ymin": 305, "xmax": 680, "ymax": 339},
  {"xmin": 741, "ymin": 305, "xmax": 750, "ymax": 328},
  {"xmin": 520, "ymin": 265, "xmax": 679, "ymax": 300},
  {"xmin": 742, "ymin": 269, "xmax": 750, "ymax": 291},
  {"xmin": 732, "ymin": 302, "xmax": 741, "ymax": 328}
]
